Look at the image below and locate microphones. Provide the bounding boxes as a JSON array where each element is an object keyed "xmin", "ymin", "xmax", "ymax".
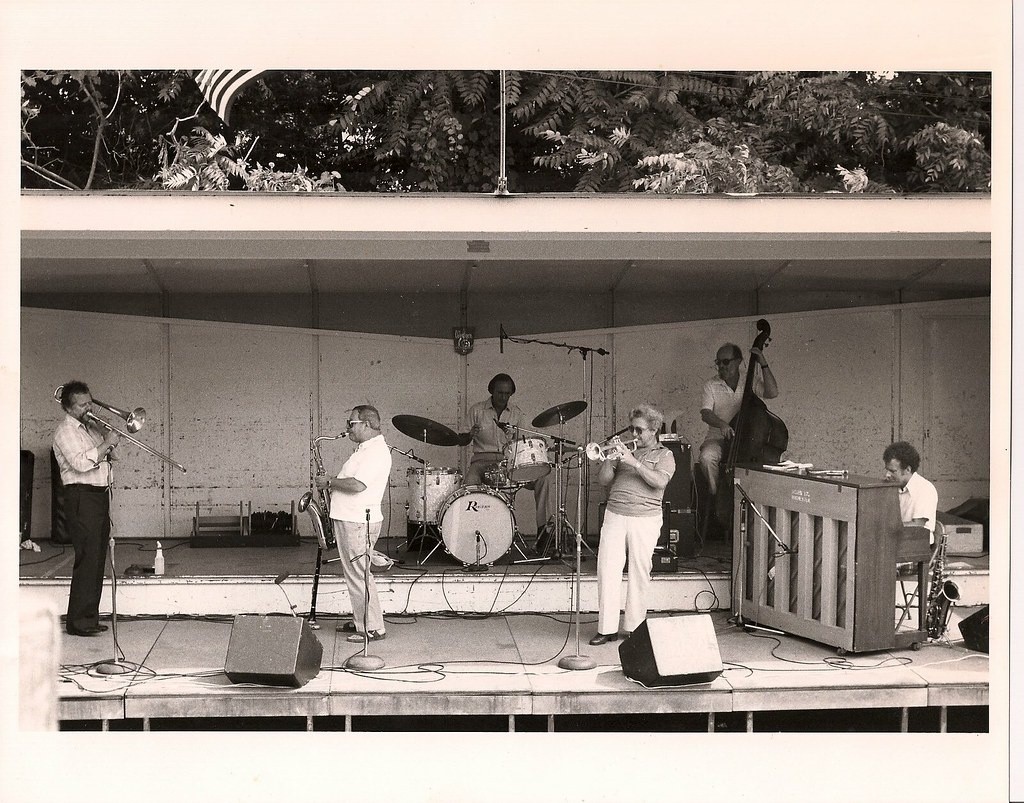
[
  {"xmin": 413, "ymin": 456, "xmax": 429, "ymax": 465},
  {"xmin": 775, "ymin": 549, "xmax": 798, "ymax": 554},
  {"xmin": 499, "ymin": 327, "xmax": 503, "ymax": 353},
  {"xmin": 104, "ymin": 444, "xmax": 116, "ymax": 456},
  {"xmin": 493, "ymin": 418, "xmax": 509, "ymax": 434}
]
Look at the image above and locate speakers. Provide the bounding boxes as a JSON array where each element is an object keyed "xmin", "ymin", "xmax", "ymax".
[
  {"xmin": 617, "ymin": 614, "xmax": 724, "ymax": 687},
  {"xmin": 957, "ymin": 602, "xmax": 990, "ymax": 653},
  {"xmin": 224, "ymin": 615, "xmax": 323, "ymax": 688}
]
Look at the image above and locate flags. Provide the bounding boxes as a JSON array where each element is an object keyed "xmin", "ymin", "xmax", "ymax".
[{"xmin": 188, "ymin": 69, "xmax": 264, "ymax": 126}]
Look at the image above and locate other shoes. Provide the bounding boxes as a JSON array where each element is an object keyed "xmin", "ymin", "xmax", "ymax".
[{"xmin": 67, "ymin": 625, "xmax": 108, "ymax": 635}]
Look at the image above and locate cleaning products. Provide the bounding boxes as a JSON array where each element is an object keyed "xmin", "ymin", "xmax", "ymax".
[{"xmin": 154, "ymin": 541, "xmax": 165, "ymax": 575}]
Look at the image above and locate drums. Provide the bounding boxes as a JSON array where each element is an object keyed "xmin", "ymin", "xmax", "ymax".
[
  {"xmin": 437, "ymin": 484, "xmax": 518, "ymax": 565},
  {"xmin": 407, "ymin": 466, "xmax": 465, "ymax": 525},
  {"xmin": 501, "ymin": 435, "xmax": 552, "ymax": 483}
]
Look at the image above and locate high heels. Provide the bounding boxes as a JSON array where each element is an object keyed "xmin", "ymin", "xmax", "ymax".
[{"xmin": 589, "ymin": 632, "xmax": 618, "ymax": 645}]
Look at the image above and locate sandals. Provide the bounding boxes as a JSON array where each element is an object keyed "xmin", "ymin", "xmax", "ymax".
[
  {"xmin": 336, "ymin": 622, "xmax": 356, "ymax": 632},
  {"xmin": 347, "ymin": 629, "xmax": 386, "ymax": 642}
]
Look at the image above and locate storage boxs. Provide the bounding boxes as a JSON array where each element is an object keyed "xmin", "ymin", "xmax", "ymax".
[{"xmin": 936, "ymin": 515, "xmax": 983, "ymax": 553}]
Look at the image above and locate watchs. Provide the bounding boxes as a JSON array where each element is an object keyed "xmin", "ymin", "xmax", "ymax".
[{"xmin": 327, "ymin": 479, "xmax": 332, "ymax": 488}]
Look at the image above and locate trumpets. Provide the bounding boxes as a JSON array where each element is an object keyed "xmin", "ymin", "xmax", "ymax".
[{"xmin": 585, "ymin": 435, "xmax": 638, "ymax": 462}]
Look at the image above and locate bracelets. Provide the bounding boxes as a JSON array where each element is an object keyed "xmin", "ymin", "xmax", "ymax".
[{"xmin": 762, "ymin": 365, "xmax": 768, "ymax": 368}]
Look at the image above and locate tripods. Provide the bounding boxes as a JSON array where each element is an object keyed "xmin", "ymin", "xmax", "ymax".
[
  {"xmin": 716, "ymin": 483, "xmax": 790, "ymax": 637},
  {"xmin": 499, "ymin": 412, "xmax": 598, "ymax": 570},
  {"xmin": 398, "ymin": 430, "xmax": 445, "ymax": 565}
]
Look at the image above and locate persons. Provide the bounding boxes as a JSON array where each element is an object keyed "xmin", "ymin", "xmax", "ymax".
[
  {"xmin": 590, "ymin": 407, "xmax": 676, "ymax": 644},
  {"xmin": 315, "ymin": 405, "xmax": 392, "ymax": 643},
  {"xmin": 698, "ymin": 345, "xmax": 778, "ymax": 515},
  {"xmin": 53, "ymin": 382, "xmax": 121, "ymax": 635},
  {"xmin": 884, "ymin": 442, "xmax": 938, "ymax": 544},
  {"xmin": 456, "ymin": 374, "xmax": 553, "ymax": 547}
]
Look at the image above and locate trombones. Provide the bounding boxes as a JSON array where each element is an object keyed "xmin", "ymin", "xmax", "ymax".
[{"xmin": 54, "ymin": 384, "xmax": 189, "ymax": 475}]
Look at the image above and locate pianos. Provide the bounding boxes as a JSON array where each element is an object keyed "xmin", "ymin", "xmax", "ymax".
[{"xmin": 731, "ymin": 459, "xmax": 934, "ymax": 656}]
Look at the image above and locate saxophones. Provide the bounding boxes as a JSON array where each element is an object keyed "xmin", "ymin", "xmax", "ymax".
[
  {"xmin": 296, "ymin": 431, "xmax": 352, "ymax": 553},
  {"xmin": 925, "ymin": 520, "xmax": 961, "ymax": 640}
]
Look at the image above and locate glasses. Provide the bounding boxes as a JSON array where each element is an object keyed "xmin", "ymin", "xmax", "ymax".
[
  {"xmin": 714, "ymin": 357, "xmax": 739, "ymax": 365},
  {"xmin": 629, "ymin": 425, "xmax": 650, "ymax": 435},
  {"xmin": 346, "ymin": 420, "xmax": 370, "ymax": 429}
]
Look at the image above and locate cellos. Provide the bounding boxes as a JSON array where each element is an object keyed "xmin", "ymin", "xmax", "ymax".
[{"xmin": 715, "ymin": 318, "xmax": 790, "ymax": 564}]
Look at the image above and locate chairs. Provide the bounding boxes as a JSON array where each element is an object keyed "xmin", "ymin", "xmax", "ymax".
[{"xmin": 894, "ymin": 519, "xmax": 945, "ymax": 631}]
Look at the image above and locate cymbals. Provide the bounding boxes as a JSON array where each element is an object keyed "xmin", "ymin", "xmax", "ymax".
[
  {"xmin": 531, "ymin": 401, "xmax": 588, "ymax": 428},
  {"xmin": 391, "ymin": 414, "xmax": 460, "ymax": 446}
]
